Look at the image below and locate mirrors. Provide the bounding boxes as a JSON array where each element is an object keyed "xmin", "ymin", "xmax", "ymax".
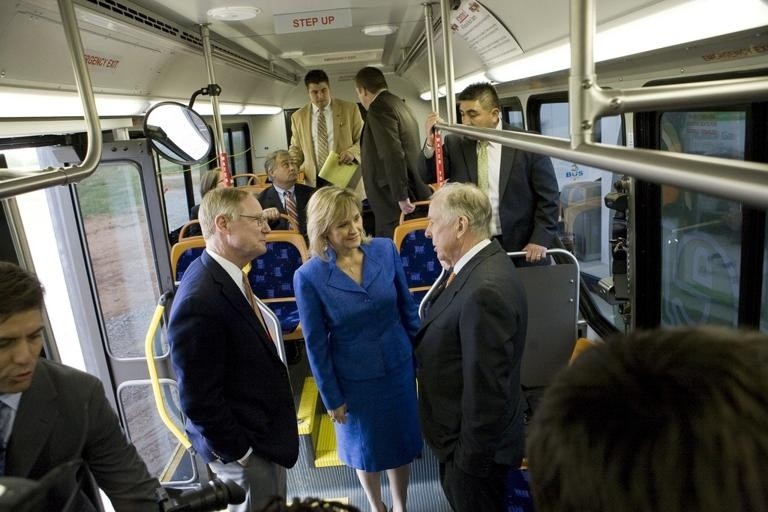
[{"xmin": 141, "ymin": 101, "xmax": 213, "ymax": 166}]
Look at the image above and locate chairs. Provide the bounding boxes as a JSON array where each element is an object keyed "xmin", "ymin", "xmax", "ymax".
[
  {"xmin": 508, "ymin": 248, "xmax": 580, "ymax": 471},
  {"xmin": 565, "ymin": 181, "xmax": 601, "ymax": 260},
  {"xmin": 393, "ymin": 200, "xmax": 443, "ymax": 308},
  {"xmin": 231, "ymin": 174, "xmax": 269, "ymax": 196},
  {"xmin": 246, "ymin": 212, "xmax": 310, "ymax": 341},
  {"xmin": 170, "ymin": 219, "xmax": 206, "ymax": 287},
  {"xmin": 247, "ymin": 172, "xmax": 272, "ymax": 186}
]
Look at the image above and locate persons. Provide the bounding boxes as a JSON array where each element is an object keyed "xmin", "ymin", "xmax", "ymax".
[
  {"xmin": 166, "ymin": 186, "xmax": 299, "ymax": 511},
  {"xmin": 354, "ymin": 67, "xmax": 433, "ymax": 239},
  {"xmin": 412, "ymin": 182, "xmax": 529, "ymax": 512},
  {"xmin": 524, "ymin": 325, "xmax": 768, "ymax": 512},
  {"xmin": 293, "ymin": 185, "xmax": 421, "ymax": 512},
  {"xmin": 416, "ymin": 83, "xmax": 560, "ymax": 267},
  {"xmin": 189, "ymin": 167, "xmax": 224, "ymax": 236},
  {"xmin": 0, "ymin": 261, "xmax": 161, "ymax": 512},
  {"xmin": 287, "ymin": 69, "xmax": 365, "ymax": 189},
  {"xmin": 256, "ymin": 150, "xmax": 318, "ymax": 250}
]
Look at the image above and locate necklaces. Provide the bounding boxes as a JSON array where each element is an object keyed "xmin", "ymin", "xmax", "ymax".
[{"xmin": 343, "ymin": 261, "xmax": 354, "ymax": 273}]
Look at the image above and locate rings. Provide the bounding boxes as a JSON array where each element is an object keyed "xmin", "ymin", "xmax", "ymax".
[
  {"xmin": 332, "ymin": 419, "xmax": 336, "ymax": 422},
  {"xmin": 537, "ymin": 255, "xmax": 541, "ymax": 257}
]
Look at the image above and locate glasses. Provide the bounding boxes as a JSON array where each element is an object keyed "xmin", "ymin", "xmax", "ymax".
[{"xmin": 237, "ymin": 213, "xmax": 267, "ymax": 226}]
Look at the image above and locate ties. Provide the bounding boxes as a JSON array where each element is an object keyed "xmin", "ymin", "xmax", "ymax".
[
  {"xmin": 243, "ymin": 270, "xmax": 270, "ymax": 339},
  {"xmin": 318, "ymin": 108, "xmax": 328, "ymax": 173},
  {"xmin": 446, "ymin": 272, "xmax": 456, "ymax": 288},
  {"xmin": 478, "ymin": 140, "xmax": 489, "ymax": 192},
  {"xmin": 285, "ymin": 192, "xmax": 300, "ymax": 231}
]
